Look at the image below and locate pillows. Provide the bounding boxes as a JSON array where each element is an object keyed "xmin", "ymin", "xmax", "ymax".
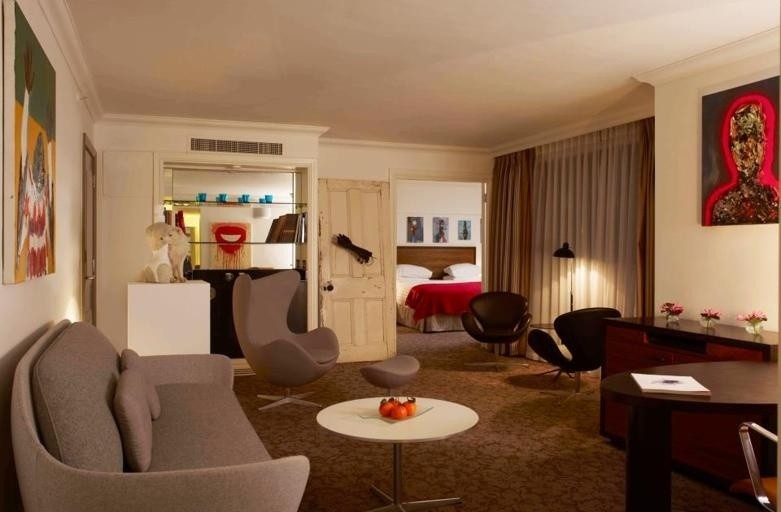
[
  {"xmin": 399, "ymin": 276, "xmax": 430, "ymax": 282},
  {"xmin": 30, "ymin": 320, "xmax": 127, "ymax": 476},
  {"xmin": 109, "ymin": 368, "xmax": 155, "ymax": 475},
  {"xmin": 443, "ymin": 262, "xmax": 482, "ymax": 278},
  {"xmin": 118, "ymin": 347, "xmax": 161, "ymax": 421},
  {"xmin": 443, "ymin": 275, "xmax": 459, "ymax": 281},
  {"xmin": 397, "ymin": 264, "xmax": 434, "ymax": 278}
]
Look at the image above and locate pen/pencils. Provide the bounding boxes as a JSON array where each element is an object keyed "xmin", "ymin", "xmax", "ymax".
[{"xmin": 652, "ymin": 380, "xmax": 678, "ymax": 384}]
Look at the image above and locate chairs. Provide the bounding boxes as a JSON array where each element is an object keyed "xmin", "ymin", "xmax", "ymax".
[
  {"xmin": 527, "ymin": 306, "xmax": 624, "ymax": 393},
  {"xmin": 459, "ymin": 291, "xmax": 534, "ymax": 379},
  {"xmin": 230, "ymin": 268, "xmax": 341, "ymax": 414},
  {"xmin": 729, "ymin": 419, "xmax": 781, "ymax": 512}
]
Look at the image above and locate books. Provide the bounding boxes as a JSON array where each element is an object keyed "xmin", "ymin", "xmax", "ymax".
[{"xmin": 630, "ymin": 372, "xmax": 712, "ymax": 396}]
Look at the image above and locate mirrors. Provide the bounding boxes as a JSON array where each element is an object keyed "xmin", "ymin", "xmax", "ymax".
[{"xmin": 160, "ymin": 169, "xmax": 302, "ymax": 270}]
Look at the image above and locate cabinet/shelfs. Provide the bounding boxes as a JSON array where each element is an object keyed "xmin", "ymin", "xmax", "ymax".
[
  {"xmin": 160, "ymin": 198, "xmax": 309, "ymax": 246},
  {"xmin": 596, "ymin": 313, "xmax": 780, "ymax": 512}
]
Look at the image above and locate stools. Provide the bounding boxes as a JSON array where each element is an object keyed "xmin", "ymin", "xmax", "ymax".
[{"xmin": 360, "ymin": 351, "xmax": 422, "ymax": 399}]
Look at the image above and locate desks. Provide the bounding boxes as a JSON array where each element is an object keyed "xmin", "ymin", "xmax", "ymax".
[
  {"xmin": 127, "ymin": 279, "xmax": 212, "ymax": 358},
  {"xmin": 529, "ymin": 322, "xmax": 577, "ymax": 385},
  {"xmin": 597, "ymin": 357, "xmax": 781, "ymax": 512}
]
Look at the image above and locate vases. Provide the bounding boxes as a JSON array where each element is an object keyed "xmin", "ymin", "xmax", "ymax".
[
  {"xmin": 666, "ymin": 314, "xmax": 680, "ymax": 323},
  {"xmin": 700, "ymin": 316, "xmax": 718, "ymax": 328},
  {"xmin": 745, "ymin": 320, "xmax": 762, "ymax": 334}
]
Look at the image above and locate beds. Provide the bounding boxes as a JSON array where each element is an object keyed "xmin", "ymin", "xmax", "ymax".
[{"xmin": 393, "ymin": 245, "xmax": 484, "ymax": 335}]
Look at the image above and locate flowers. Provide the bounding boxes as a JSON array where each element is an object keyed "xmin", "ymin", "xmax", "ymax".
[
  {"xmin": 699, "ymin": 306, "xmax": 723, "ymax": 329},
  {"xmin": 659, "ymin": 300, "xmax": 684, "ymax": 316},
  {"xmin": 734, "ymin": 309, "xmax": 769, "ymax": 333}
]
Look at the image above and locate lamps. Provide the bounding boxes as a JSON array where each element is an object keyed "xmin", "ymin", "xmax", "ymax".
[
  {"xmin": 251, "ymin": 205, "xmax": 272, "ymax": 220},
  {"xmin": 552, "ymin": 242, "xmax": 578, "ymax": 313}
]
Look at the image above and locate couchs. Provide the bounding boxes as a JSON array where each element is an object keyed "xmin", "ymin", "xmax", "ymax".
[{"xmin": 7, "ymin": 317, "xmax": 314, "ymax": 512}]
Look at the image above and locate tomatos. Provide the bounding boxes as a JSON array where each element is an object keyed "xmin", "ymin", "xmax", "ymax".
[
  {"xmin": 388, "ymin": 395, "xmax": 401, "ymax": 408},
  {"xmin": 403, "ymin": 391, "xmax": 417, "ymax": 416},
  {"xmin": 390, "ymin": 405, "xmax": 407, "ymax": 420},
  {"xmin": 379, "ymin": 398, "xmax": 394, "ymax": 416}
]
